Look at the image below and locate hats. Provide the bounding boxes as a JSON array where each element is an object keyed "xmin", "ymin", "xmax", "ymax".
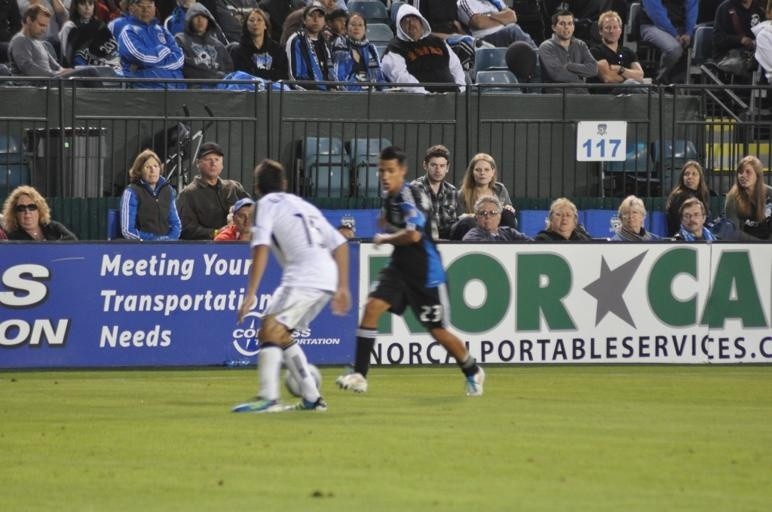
[
  {"xmin": 304, "ymin": 2, "xmax": 326, "ymax": 17},
  {"xmin": 235, "ymin": 199, "xmax": 255, "ymax": 213},
  {"xmin": 198, "ymin": 142, "xmax": 224, "ymax": 161}
]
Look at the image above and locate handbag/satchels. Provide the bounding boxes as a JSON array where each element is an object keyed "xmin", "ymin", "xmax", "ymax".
[{"xmin": 743, "ymin": 46, "xmax": 759, "ymax": 71}]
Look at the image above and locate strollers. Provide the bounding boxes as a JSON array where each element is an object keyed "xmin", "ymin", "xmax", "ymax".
[{"xmin": 116, "ymin": 102, "xmax": 214, "ymax": 191}]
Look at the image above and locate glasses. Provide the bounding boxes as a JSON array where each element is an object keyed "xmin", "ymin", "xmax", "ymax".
[
  {"xmin": 17, "ymin": 204, "xmax": 37, "ymax": 212},
  {"xmin": 478, "ymin": 210, "xmax": 499, "ymax": 217},
  {"xmin": 36, "ymin": 18, "xmax": 47, "ymax": 30}
]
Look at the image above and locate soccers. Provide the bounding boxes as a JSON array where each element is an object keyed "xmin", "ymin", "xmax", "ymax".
[{"xmin": 285, "ymin": 364, "xmax": 322, "ymax": 398}]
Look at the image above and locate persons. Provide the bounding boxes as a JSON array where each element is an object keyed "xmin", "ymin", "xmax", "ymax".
[
  {"xmin": 665, "ymin": 156, "xmax": 771, "ymax": 242},
  {"xmin": 610, "ymin": 196, "xmax": 661, "ymax": 242},
  {"xmin": 232, "ymin": 158, "xmax": 352, "ymax": 414},
  {"xmin": 336, "ymin": 145, "xmax": 486, "ymax": 397},
  {"xmin": 530, "ymin": 196, "xmax": 591, "ymax": 241},
  {"xmin": 2, "ymin": 1, "xmax": 771, "ymax": 91},
  {"xmin": 339, "ymin": 147, "xmax": 523, "ymax": 241},
  {"xmin": 120, "ymin": 142, "xmax": 255, "ymax": 240},
  {"xmin": 1, "ymin": 186, "xmax": 78, "ymax": 242}
]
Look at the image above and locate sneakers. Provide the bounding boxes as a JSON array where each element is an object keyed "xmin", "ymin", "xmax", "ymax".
[
  {"xmin": 233, "ymin": 396, "xmax": 283, "ymax": 414},
  {"xmin": 336, "ymin": 373, "xmax": 368, "ymax": 394},
  {"xmin": 286, "ymin": 397, "xmax": 327, "ymax": 411},
  {"xmin": 465, "ymin": 366, "xmax": 485, "ymax": 397}
]
